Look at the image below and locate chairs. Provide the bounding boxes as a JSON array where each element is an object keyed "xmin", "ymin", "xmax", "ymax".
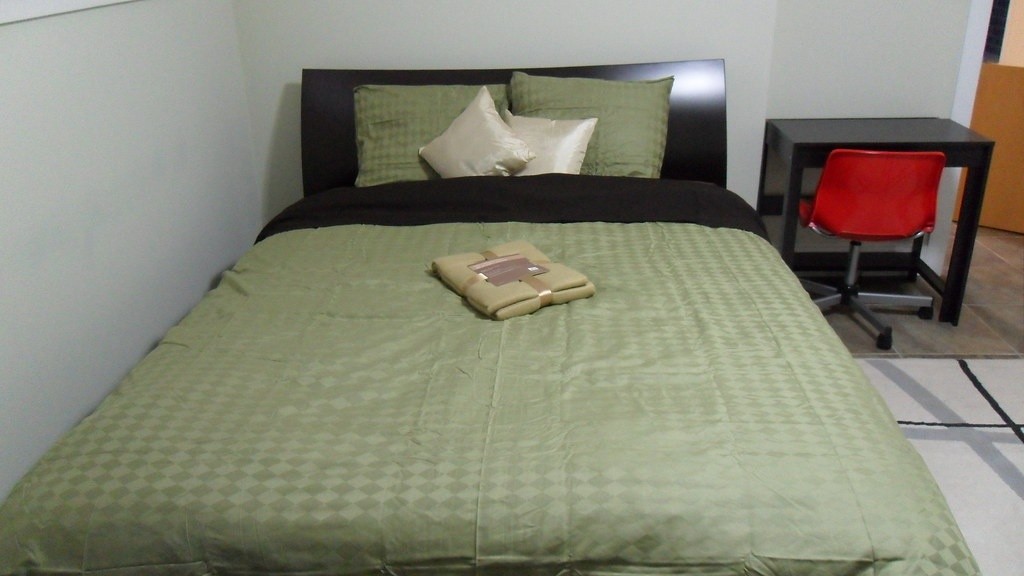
[{"xmin": 790, "ymin": 149, "xmax": 948, "ymax": 351}]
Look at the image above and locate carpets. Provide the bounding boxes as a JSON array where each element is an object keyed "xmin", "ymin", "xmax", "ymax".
[{"xmin": 856, "ymin": 356, "xmax": 1024, "ymax": 576}]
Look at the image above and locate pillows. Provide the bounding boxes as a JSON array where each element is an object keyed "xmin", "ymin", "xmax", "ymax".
[
  {"xmin": 418, "ymin": 86, "xmax": 536, "ymax": 179},
  {"xmin": 353, "ymin": 83, "xmax": 512, "ymax": 188},
  {"xmin": 511, "ymin": 72, "xmax": 675, "ymax": 180},
  {"xmin": 504, "ymin": 109, "xmax": 601, "ymax": 176}
]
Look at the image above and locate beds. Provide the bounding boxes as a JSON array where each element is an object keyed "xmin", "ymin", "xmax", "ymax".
[{"xmin": 0, "ymin": 58, "xmax": 991, "ymax": 576}]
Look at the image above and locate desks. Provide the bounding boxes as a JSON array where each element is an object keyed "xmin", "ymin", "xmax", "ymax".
[{"xmin": 755, "ymin": 116, "xmax": 996, "ymax": 328}]
